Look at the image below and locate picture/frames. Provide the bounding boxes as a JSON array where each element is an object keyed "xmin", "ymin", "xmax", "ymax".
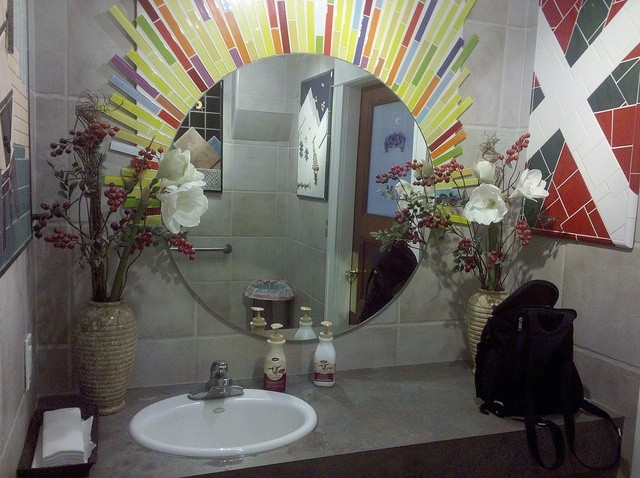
[
  {"xmin": 170, "ymin": 79, "xmax": 224, "ymax": 193},
  {"xmin": 521, "ymin": 0, "xmax": 640, "ymax": 249},
  {"xmin": 296, "ymin": 69, "xmax": 334, "ymax": 203},
  {"xmin": 0, "ymin": 26, "xmax": 33, "ymax": 276}
]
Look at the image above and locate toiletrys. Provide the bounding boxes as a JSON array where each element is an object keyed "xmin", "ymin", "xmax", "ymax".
[
  {"xmin": 248, "ymin": 306, "xmax": 267, "ymax": 337},
  {"xmin": 263, "ymin": 322, "xmax": 287, "ymax": 391}
]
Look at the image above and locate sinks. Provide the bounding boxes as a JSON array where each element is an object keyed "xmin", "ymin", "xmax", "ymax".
[{"xmin": 128, "ymin": 388, "xmax": 319, "ymax": 456}]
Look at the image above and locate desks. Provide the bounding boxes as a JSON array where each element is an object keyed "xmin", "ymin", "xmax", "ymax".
[{"xmin": 15, "ymin": 359, "xmax": 625, "ymax": 478}]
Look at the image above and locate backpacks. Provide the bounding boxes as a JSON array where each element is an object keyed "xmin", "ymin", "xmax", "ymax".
[{"xmin": 473, "ymin": 278, "xmax": 627, "ymax": 471}]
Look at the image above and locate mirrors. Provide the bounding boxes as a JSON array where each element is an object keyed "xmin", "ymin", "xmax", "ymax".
[{"xmin": 160, "ymin": 52, "xmax": 435, "ymax": 342}]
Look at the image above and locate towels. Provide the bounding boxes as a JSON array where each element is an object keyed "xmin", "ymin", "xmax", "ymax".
[{"xmin": 42, "ymin": 407, "xmax": 86, "ymax": 467}]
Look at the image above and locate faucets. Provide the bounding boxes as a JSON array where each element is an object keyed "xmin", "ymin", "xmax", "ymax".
[{"xmin": 188, "ymin": 359, "xmax": 244, "ymax": 400}]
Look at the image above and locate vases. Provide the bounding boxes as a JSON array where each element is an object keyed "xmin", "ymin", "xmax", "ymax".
[
  {"xmin": 70, "ymin": 303, "xmax": 138, "ymax": 416},
  {"xmin": 467, "ymin": 291, "xmax": 512, "ymax": 373}
]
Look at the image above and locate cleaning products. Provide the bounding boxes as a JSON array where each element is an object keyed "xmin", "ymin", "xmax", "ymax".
[
  {"xmin": 313, "ymin": 319, "xmax": 336, "ymax": 388},
  {"xmin": 294, "ymin": 305, "xmax": 316, "ymax": 341}
]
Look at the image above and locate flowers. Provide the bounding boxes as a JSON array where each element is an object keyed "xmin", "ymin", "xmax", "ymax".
[
  {"xmin": 33, "ymin": 89, "xmax": 208, "ymax": 302},
  {"xmin": 371, "ymin": 133, "xmax": 557, "ymax": 291}
]
